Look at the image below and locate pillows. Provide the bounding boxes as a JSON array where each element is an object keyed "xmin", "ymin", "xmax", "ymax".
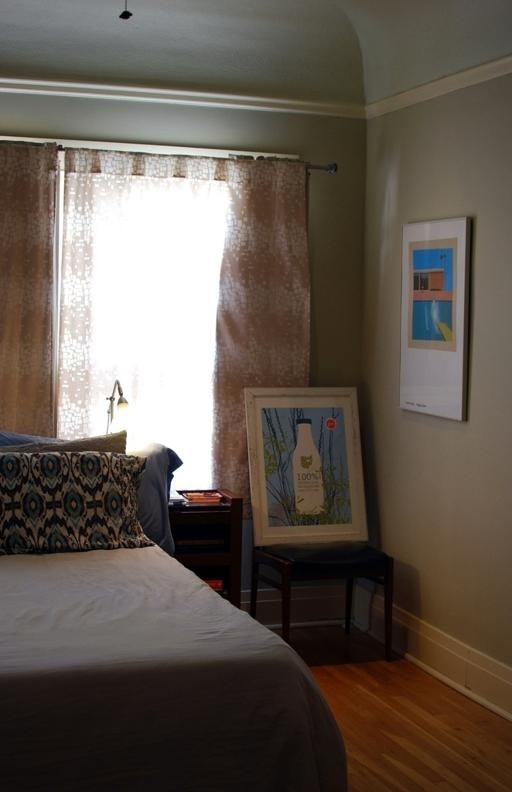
[
  {"xmin": 0, "ymin": 430, "xmax": 183, "ymax": 557},
  {"xmin": 37, "ymin": 430, "xmax": 127, "ymax": 454},
  {"xmin": 0, "ymin": 451, "xmax": 156, "ymax": 555}
]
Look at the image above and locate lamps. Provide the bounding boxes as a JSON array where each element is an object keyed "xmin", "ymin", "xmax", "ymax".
[{"xmin": 104, "ymin": 380, "xmax": 128, "ymax": 436}]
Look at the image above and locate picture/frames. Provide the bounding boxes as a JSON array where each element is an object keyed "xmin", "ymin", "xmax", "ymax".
[
  {"xmin": 244, "ymin": 386, "xmax": 369, "ymax": 547},
  {"xmin": 399, "ymin": 217, "xmax": 472, "ymax": 422}
]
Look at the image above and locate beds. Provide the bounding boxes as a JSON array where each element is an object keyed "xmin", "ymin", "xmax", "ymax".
[{"xmin": 0, "ymin": 431, "xmax": 347, "ymax": 791}]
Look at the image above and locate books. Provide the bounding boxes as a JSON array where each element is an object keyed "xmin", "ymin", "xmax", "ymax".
[
  {"xmin": 183, "ymin": 491, "xmax": 223, "ymax": 503},
  {"xmin": 204, "ymin": 574, "xmax": 224, "ymax": 589}
]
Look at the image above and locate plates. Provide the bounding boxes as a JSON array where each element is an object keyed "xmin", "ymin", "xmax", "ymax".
[{"xmin": 183, "ymin": 492, "xmax": 223, "ymax": 504}]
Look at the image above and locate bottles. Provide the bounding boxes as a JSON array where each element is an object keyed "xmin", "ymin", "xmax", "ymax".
[{"xmin": 291, "ymin": 418, "xmax": 325, "ymax": 516}]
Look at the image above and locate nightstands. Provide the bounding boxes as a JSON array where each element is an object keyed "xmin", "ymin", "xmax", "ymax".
[{"xmin": 168, "ymin": 488, "xmax": 243, "ymax": 609}]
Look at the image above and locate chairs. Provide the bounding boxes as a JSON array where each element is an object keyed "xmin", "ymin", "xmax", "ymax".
[{"xmin": 250, "ymin": 538, "xmax": 393, "ymax": 662}]
